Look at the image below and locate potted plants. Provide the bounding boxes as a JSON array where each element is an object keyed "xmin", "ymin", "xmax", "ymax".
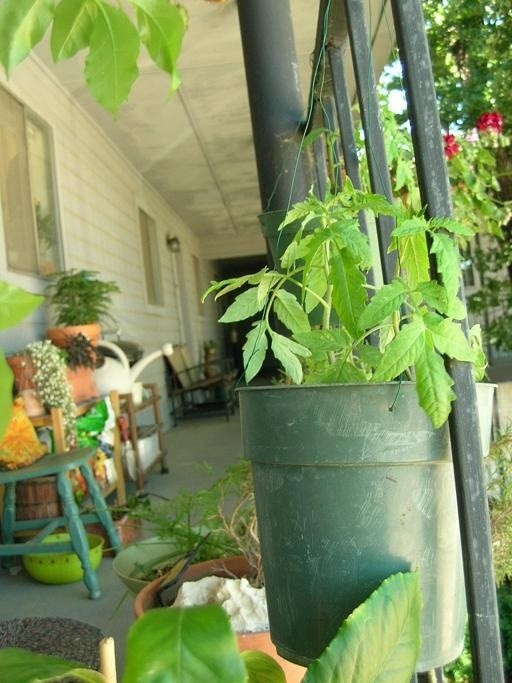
[
  {"xmin": 6, "ymin": 338, "xmax": 71, "ymax": 408},
  {"xmin": 202, "ymin": 174, "xmax": 499, "ymax": 675},
  {"xmin": 49, "ymin": 265, "xmax": 118, "ymax": 359},
  {"xmin": 133, "ymin": 492, "xmax": 305, "ymax": 683},
  {"xmin": 111, "ymin": 460, "xmax": 255, "ymax": 594}
]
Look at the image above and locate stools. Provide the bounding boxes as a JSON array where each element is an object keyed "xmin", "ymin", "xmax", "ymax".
[{"xmin": 1, "ymin": 447, "xmax": 126, "ymax": 601}]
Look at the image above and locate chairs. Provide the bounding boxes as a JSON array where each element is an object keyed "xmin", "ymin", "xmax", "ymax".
[{"xmin": 162, "ymin": 342, "xmax": 238, "ymax": 428}]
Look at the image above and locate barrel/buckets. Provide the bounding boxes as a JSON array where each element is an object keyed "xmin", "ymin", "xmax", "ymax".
[
  {"xmin": 232, "ymin": 380, "xmax": 499, "ymax": 672},
  {"xmin": 15, "ymin": 476, "xmax": 59, "ymax": 521}
]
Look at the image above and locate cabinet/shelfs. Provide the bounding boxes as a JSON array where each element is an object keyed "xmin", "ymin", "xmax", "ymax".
[
  {"xmin": 119, "ymin": 383, "xmax": 170, "ymax": 488},
  {"xmin": 14, "ymin": 387, "xmax": 126, "ymax": 537}
]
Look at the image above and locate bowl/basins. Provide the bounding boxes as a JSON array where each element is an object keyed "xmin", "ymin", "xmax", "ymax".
[{"xmin": 23, "ymin": 533, "xmax": 104, "ymax": 585}]
